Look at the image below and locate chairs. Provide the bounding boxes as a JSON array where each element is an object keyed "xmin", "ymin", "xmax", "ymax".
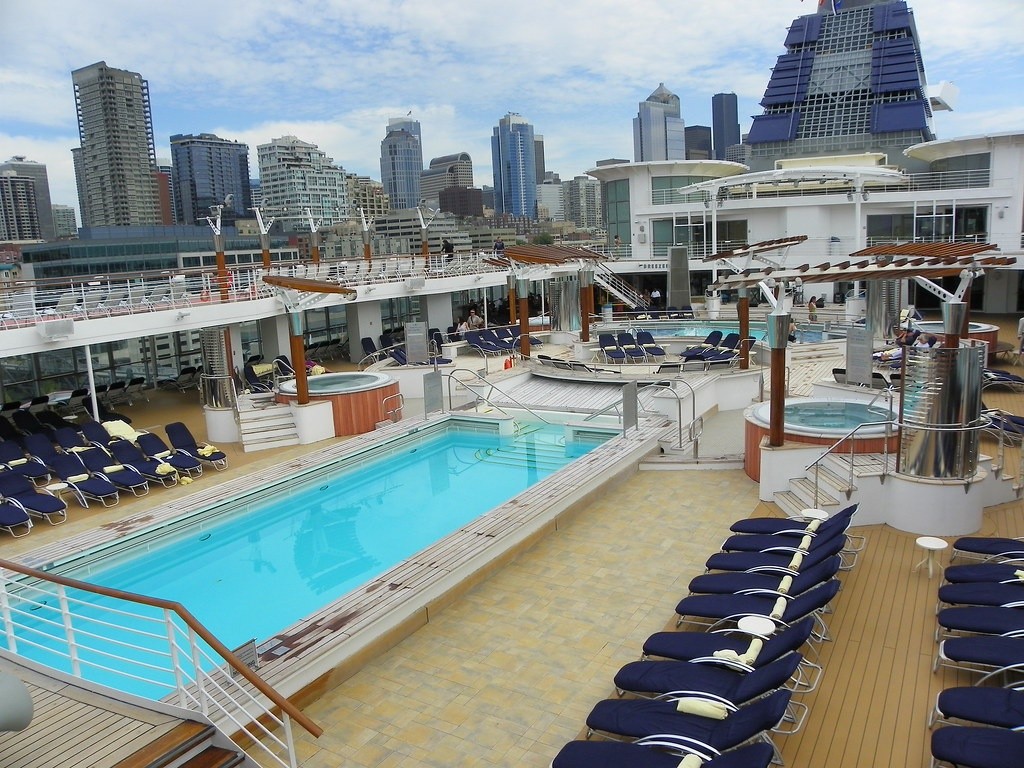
[
  {"xmin": 0, "ymin": 377, "xmax": 154, "ymax": 450},
  {"xmin": 980, "ymin": 400, "xmax": 1024, "ymax": 448},
  {"xmin": 873, "ymin": 330, "xmax": 938, "ymax": 369},
  {"xmin": 538, "ymin": 355, "xmax": 709, "ymax": 374},
  {"xmin": 464, "ymin": 326, "xmax": 543, "ymax": 357},
  {"xmin": 549, "ymin": 502, "xmax": 866, "ymax": 768},
  {"xmin": 679, "ymin": 330, "xmax": 756, "ymax": 372},
  {"xmin": 928, "ymin": 536, "xmax": 1024, "ymax": 768},
  {"xmin": 832, "ymin": 368, "xmax": 901, "ymax": 393},
  {"xmin": 634, "ymin": 306, "xmax": 694, "ymax": 320},
  {"xmin": 981, "ymin": 367, "xmax": 1024, "ymax": 393},
  {"xmin": 156, "ymin": 338, "xmax": 351, "ymax": 395},
  {"xmin": 900, "ymin": 309, "xmax": 910, "ymax": 324},
  {"xmin": 361, "ymin": 325, "xmax": 465, "ymax": 363},
  {"xmin": 598, "ymin": 332, "xmax": 666, "ymax": 364},
  {"xmin": 0, "ymin": 254, "xmax": 484, "ymax": 330},
  {"xmin": 0, "ymin": 420, "xmax": 228, "ymax": 538},
  {"xmin": 389, "ymin": 347, "xmax": 452, "ymax": 365},
  {"xmin": 1011, "ymin": 337, "xmax": 1024, "ymax": 366}
]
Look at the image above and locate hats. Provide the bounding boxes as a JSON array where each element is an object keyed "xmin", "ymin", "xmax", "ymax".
[{"xmin": 908, "ymin": 327, "xmax": 916, "ymax": 334}]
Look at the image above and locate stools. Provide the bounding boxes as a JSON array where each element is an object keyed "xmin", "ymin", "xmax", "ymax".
[
  {"xmin": 659, "ymin": 343, "xmax": 671, "ymax": 361},
  {"xmin": 589, "ymin": 348, "xmax": 602, "ymax": 362},
  {"xmin": 912, "ymin": 536, "xmax": 949, "ymax": 579}
]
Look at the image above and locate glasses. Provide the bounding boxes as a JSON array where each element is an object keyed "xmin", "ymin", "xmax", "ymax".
[{"xmin": 471, "ymin": 312, "xmax": 475, "ymax": 314}]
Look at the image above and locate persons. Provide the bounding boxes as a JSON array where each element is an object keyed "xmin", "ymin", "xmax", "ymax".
[
  {"xmin": 898, "ymin": 328, "xmax": 937, "ymax": 347},
  {"xmin": 809, "ymin": 296, "xmax": 817, "ymax": 321},
  {"xmin": 1017, "ymin": 317, "xmax": 1024, "ymax": 354},
  {"xmin": 441, "ymin": 239, "xmax": 454, "ymax": 262},
  {"xmin": 788, "ymin": 318, "xmax": 796, "ymax": 341},
  {"xmin": 644, "ymin": 288, "xmax": 661, "ymax": 305},
  {"xmin": 455, "ymin": 299, "xmax": 479, "ymax": 340},
  {"xmin": 494, "ymin": 237, "xmax": 504, "ymax": 250},
  {"xmin": 614, "ymin": 234, "xmax": 620, "ymax": 247}
]
